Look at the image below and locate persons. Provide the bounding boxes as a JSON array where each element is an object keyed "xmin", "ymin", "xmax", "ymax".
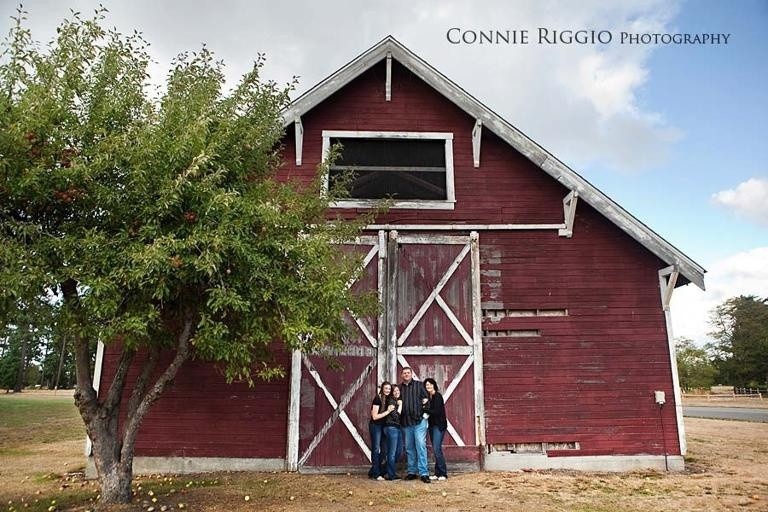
[{"xmin": 368, "ymin": 367, "xmax": 447, "ymax": 483}]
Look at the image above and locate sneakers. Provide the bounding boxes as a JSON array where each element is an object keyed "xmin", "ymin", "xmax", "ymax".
[
  {"xmin": 429, "ymin": 475, "xmax": 446, "ymax": 481},
  {"xmin": 405, "ymin": 474, "xmax": 429, "ymax": 483},
  {"xmin": 375, "ymin": 473, "xmax": 402, "ymax": 481}
]
